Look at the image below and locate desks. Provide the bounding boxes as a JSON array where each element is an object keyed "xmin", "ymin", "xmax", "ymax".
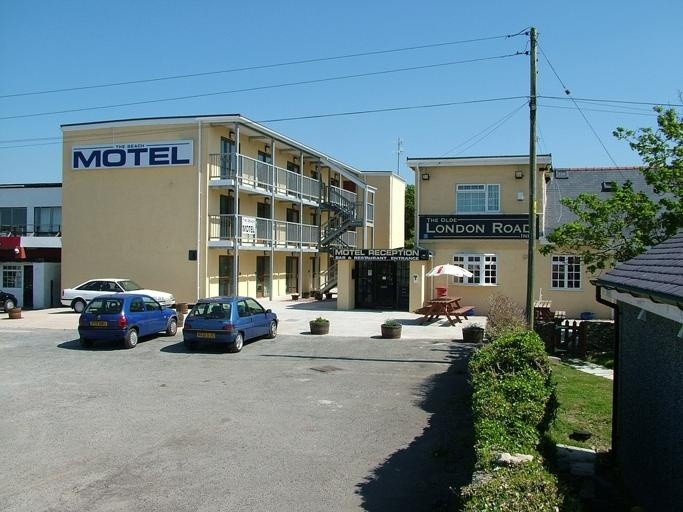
[
  {"xmin": 417, "ymin": 296, "xmax": 468, "ymax": 327},
  {"xmin": 534, "ymin": 300, "xmax": 553, "ymax": 322}
]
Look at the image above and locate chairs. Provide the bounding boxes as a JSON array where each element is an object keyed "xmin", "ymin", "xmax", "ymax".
[
  {"xmin": 115, "ymin": 284, "xmax": 122, "ymax": 292},
  {"xmin": 107, "ymin": 284, "xmax": 111, "ymax": 291},
  {"xmin": 238, "ymin": 306, "xmax": 249, "ymax": 317},
  {"xmin": 210, "ymin": 306, "xmax": 225, "ymax": 318},
  {"xmin": 131, "ymin": 303, "xmax": 140, "ymax": 311}
]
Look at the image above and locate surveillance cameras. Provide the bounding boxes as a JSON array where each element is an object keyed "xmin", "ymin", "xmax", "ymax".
[{"xmin": 14, "ymin": 249, "xmax": 19, "ymax": 255}]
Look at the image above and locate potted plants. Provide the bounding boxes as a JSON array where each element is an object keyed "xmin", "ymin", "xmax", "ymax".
[
  {"xmin": 381, "ymin": 319, "xmax": 402, "ymax": 339},
  {"xmin": 9, "ymin": 307, "xmax": 21, "ymax": 318},
  {"xmin": 309, "ymin": 317, "xmax": 330, "ymax": 334},
  {"xmin": 462, "ymin": 323, "xmax": 484, "ymax": 343}
]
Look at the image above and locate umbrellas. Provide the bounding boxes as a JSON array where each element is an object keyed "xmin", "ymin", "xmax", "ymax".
[{"xmin": 423, "ymin": 262, "xmax": 474, "ymax": 313}]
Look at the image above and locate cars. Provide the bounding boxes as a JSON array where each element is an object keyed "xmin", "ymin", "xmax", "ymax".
[
  {"xmin": 0, "ymin": 289, "xmax": 18, "ymax": 313},
  {"xmin": 60, "ymin": 278, "xmax": 178, "ymax": 349},
  {"xmin": 182, "ymin": 295, "xmax": 279, "ymax": 352}
]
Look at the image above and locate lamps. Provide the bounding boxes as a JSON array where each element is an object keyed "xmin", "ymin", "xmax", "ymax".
[
  {"xmin": 422, "ymin": 174, "xmax": 429, "ymax": 180},
  {"xmin": 515, "ymin": 171, "xmax": 523, "ymax": 179}
]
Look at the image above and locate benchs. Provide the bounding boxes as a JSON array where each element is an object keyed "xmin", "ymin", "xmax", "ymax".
[
  {"xmin": 450, "ymin": 306, "xmax": 476, "ymax": 315},
  {"xmin": 555, "ymin": 310, "xmax": 566, "ymax": 324},
  {"xmin": 416, "ymin": 304, "xmax": 432, "ymax": 314}
]
